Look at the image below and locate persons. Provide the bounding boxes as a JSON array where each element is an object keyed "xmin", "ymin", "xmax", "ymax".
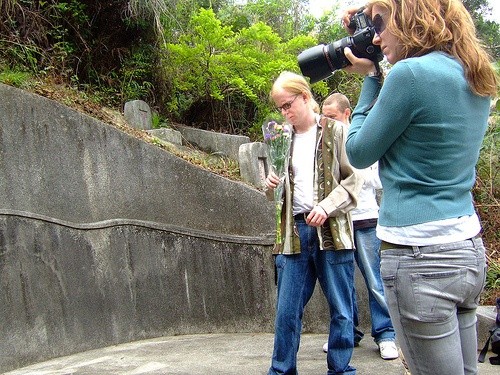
[
  {"xmin": 264, "ymin": 70, "xmax": 358, "ymax": 375},
  {"xmin": 322, "ymin": 92, "xmax": 400, "ymax": 360},
  {"xmin": 344, "ymin": 0, "xmax": 500, "ymax": 375}
]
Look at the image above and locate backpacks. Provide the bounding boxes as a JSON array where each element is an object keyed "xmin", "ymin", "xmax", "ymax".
[{"xmin": 478, "ymin": 297, "xmax": 500, "ymax": 365}]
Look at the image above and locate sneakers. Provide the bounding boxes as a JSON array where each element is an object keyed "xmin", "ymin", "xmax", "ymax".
[
  {"xmin": 323, "ymin": 343, "xmax": 328, "ymax": 352},
  {"xmin": 378, "ymin": 341, "xmax": 398, "ymax": 359}
]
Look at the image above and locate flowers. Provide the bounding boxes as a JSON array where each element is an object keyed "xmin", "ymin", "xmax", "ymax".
[{"xmin": 262, "ymin": 121, "xmax": 293, "ymax": 245}]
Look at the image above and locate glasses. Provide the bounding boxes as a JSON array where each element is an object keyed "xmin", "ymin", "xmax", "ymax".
[
  {"xmin": 372, "ymin": 14, "xmax": 386, "ymax": 35},
  {"xmin": 277, "ymin": 95, "xmax": 297, "ymax": 112}
]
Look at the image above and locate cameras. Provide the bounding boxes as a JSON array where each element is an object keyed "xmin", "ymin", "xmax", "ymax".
[{"xmin": 296, "ymin": 9, "xmax": 384, "ymax": 84}]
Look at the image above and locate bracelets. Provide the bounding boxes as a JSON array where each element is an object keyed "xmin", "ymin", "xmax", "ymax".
[{"xmin": 365, "ymin": 71, "xmax": 378, "ymax": 77}]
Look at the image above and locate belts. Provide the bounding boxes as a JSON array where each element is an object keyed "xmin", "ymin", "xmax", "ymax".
[
  {"xmin": 381, "ymin": 233, "xmax": 424, "ymax": 250},
  {"xmin": 295, "ymin": 212, "xmax": 310, "ymax": 220}
]
